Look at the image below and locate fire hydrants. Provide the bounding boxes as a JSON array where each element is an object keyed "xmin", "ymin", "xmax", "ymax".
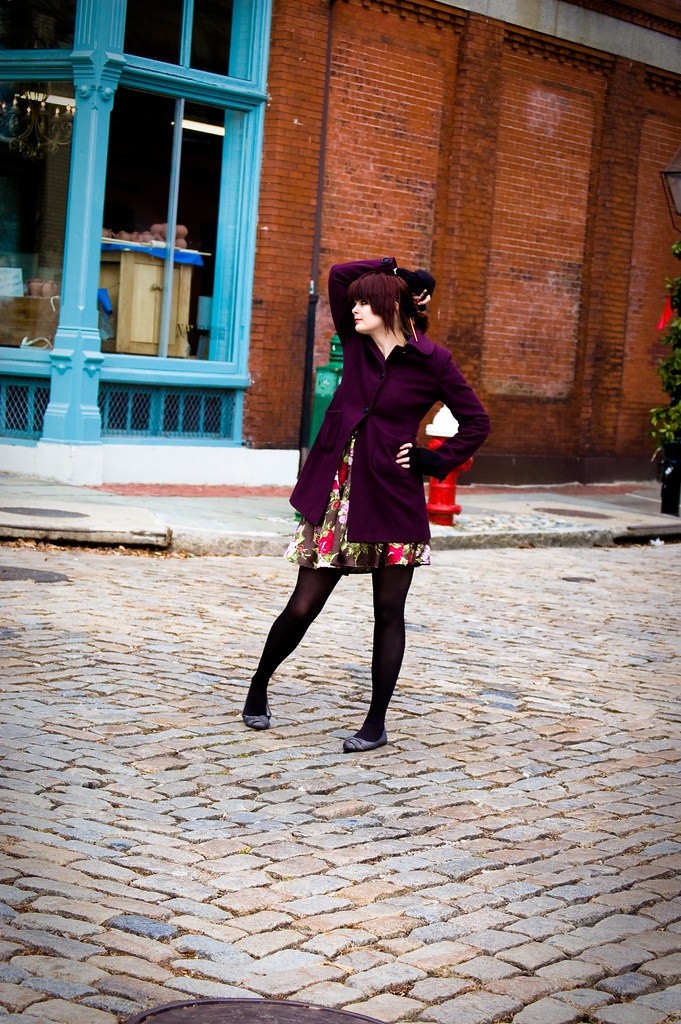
[{"xmin": 422, "ymin": 405, "xmax": 474, "ymax": 526}]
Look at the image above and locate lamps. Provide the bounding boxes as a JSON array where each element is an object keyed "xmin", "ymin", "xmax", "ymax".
[{"xmin": 0, "ymin": 0, "xmax": 75, "ymax": 160}]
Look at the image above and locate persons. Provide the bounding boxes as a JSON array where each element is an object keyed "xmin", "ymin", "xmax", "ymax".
[{"xmin": 242, "ymin": 257, "xmax": 489, "ymax": 753}]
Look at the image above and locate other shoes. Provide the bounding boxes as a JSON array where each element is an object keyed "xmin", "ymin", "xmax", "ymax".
[
  {"xmin": 344, "ymin": 727, "xmax": 387, "ymax": 751},
  {"xmin": 243, "ymin": 677, "xmax": 273, "ymax": 729}
]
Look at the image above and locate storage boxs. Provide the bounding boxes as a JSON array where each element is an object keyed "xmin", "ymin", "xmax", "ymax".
[
  {"xmin": 97, "ymin": 249, "xmax": 191, "ymax": 358},
  {"xmin": 0, "ymin": 295, "xmax": 59, "ymax": 349}
]
